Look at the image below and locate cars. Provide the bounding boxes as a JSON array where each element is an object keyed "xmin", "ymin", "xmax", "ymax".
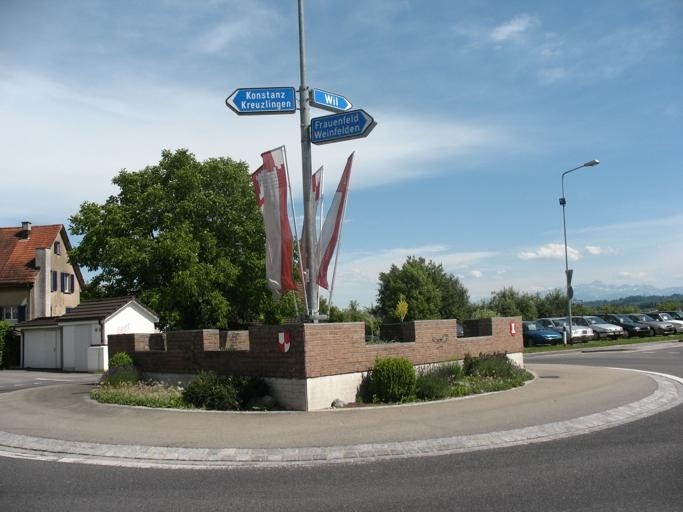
[
  {"xmin": 539, "ymin": 316, "xmax": 594, "ymax": 343},
  {"xmin": 521, "ymin": 321, "xmax": 561, "ymax": 346},
  {"xmin": 582, "ymin": 316, "xmax": 623, "ymax": 340},
  {"xmin": 605, "ymin": 314, "xmax": 650, "ymax": 337},
  {"xmin": 627, "ymin": 312, "xmax": 683, "ymax": 336}
]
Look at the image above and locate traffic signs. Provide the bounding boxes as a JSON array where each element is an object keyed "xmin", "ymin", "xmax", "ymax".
[
  {"xmin": 308, "ymin": 89, "xmax": 354, "ymax": 113},
  {"xmin": 309, "ymin": 107, "xmax": 379, "ymax": 145},
  {"xmin": 224, "ymin": 87, "xmax": 297, "ymax": 116}
]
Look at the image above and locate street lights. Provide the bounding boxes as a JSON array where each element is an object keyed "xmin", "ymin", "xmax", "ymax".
[{"xmin": 560, "ymin": 159, "xmax": 600, "ymax": 346}]
